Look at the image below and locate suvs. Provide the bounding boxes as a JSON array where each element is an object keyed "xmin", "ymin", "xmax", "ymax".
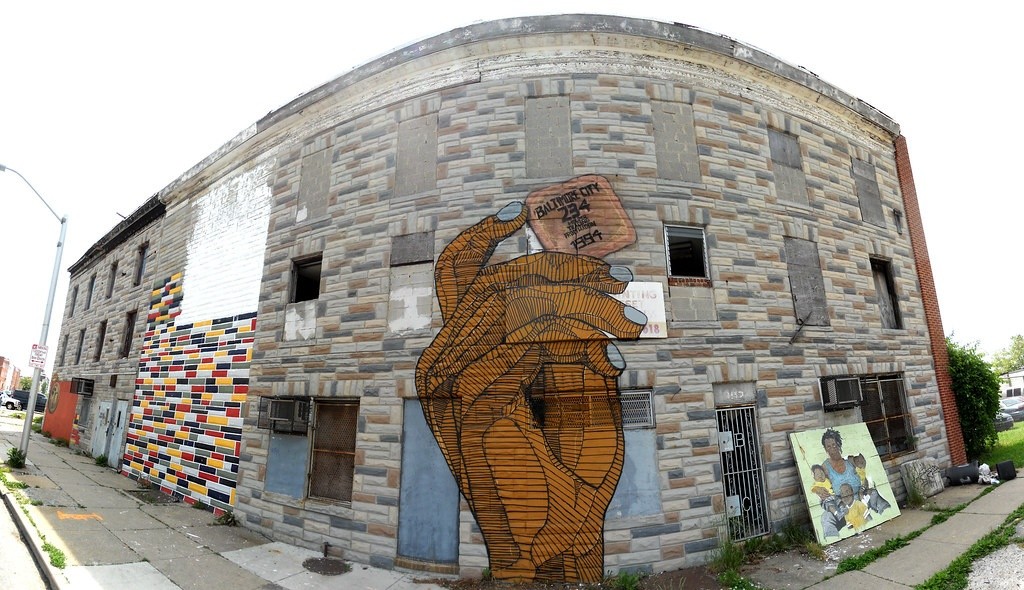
[{"xmin": 12, "ymin": 390, "xmax": 48, "ymax": 412}]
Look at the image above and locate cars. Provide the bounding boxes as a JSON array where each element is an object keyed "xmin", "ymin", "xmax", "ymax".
[
  {"xmin": 995, "ymin": 412, "xmax": 1014, "ymax": 430},
  {"xmin": 0, "ymin": 392, "xmax": 21, "ymax": 410},
  {"xmin": 997, "ymin": 395, "xmax": 1024, "ymax": 420}
]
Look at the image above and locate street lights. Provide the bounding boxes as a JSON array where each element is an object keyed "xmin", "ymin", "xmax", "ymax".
[{"xmin": 0, "ymin": 164, "xmax": 69, "ymax": 468}]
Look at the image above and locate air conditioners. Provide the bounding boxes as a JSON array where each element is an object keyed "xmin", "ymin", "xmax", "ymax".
[
  {"xmin": 268, "ymin": 400, "xmax": 309, "ymax": 422},
  {"xmin": 73, "ymin": 379, "xmax": 93, "ymax": 395},
  {"xmin": 822, "ymin": 378, "xmax": 863, "ymax": 407}
]
